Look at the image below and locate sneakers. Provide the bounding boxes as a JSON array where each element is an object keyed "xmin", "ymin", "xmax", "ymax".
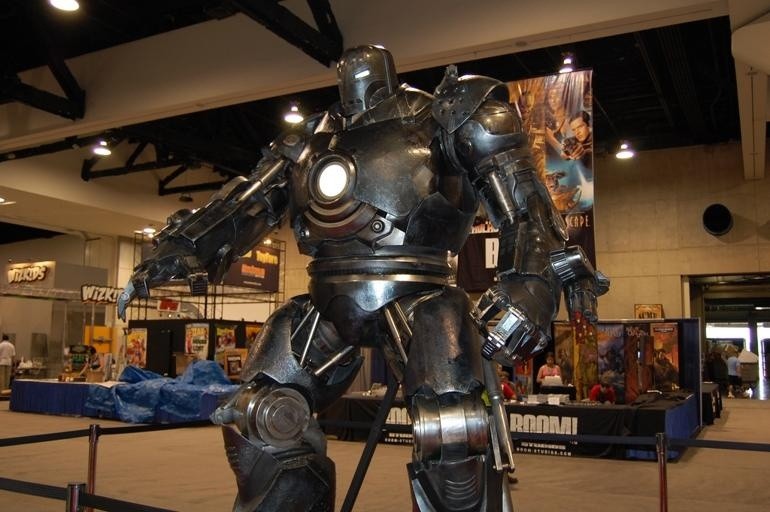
[{"xmin": 728, "ymin": 394, "xmax": 735, "ymax": 398}]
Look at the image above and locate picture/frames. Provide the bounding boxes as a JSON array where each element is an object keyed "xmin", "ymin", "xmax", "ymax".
[{"xmin": 634, "ymin": 304, "xmax": 662, "ymax": 319}]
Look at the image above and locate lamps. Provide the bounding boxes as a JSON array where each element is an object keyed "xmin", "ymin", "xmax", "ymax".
[
  {"xmin": 615, "ymin": 141, "xmax": 636, "ymax": 160},
  {"xmin": 559, "ymin": 55, "xmax": 575, "ymax": 73},
  {"xmin": 283, "ymin": 101, "xmax": 305, "ymax": 124},
  {"xmin": 93, "ymin": 139, "xmax": 112, "ymax": 157}
]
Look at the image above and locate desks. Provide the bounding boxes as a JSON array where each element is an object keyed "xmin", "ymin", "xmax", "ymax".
[
  {"xmin": 485, "ymin": 392, "xmax": 699, "ymax": 464},
  {"xmin": 9, "ymin": 378, "xmax": 91, "ymax": 417},
  {"xmin": 316, "ymin": 391, "xmax": 415, "ymax": 446},
  {"xmin": 702, "ymin": 382, "xmax": 723, "ymax": 425}
]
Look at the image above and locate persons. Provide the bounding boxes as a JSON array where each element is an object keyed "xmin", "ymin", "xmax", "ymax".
[
  {"xmin": 0, "ymin": 334, "xmax": 15, "ymax": 390},
  {"xmin": 705, "ymin": 349, "xmax": 757, "ymax": 399},
  {"xmin": 497, "ymin": 364, "xmax": 524, "ymax": 402},
  {"xmin": 654, "ymin": 350, "xmax": 678, "ymax": 389},
  {"xmin": 561, "ymin": 111, "xmax": 593, "ymax": 161},
  {"xmin": 588, "ymin": 369, "xmax": 617, "ymax": 405},
  {"xmin": 215, "ymin": 334, "xmax": 236, "ymax": 353},
  {"xmin": 185, "ymin": 330, "xmax": 194, "ymax": 354},
  {"xmin": 200, "ymin": 329, "xmax": 208, "ymax": 359},
  {"xmin": 536, "ymin": 350, "xmax": 562, "ymax": 396}
]
